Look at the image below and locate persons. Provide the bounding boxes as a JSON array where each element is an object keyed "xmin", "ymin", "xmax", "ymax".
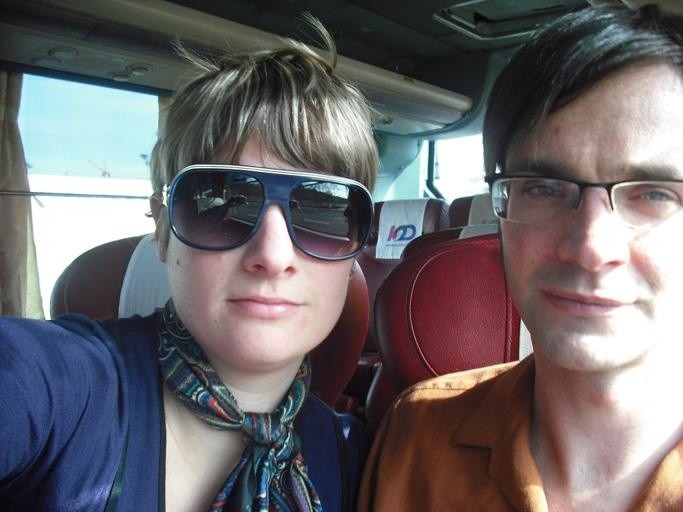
[
  {"xmin": 356, "ymin": 0, "xmax": 683, "ymax": 510},
  {"xmin": 0, "ymin": 37, "xmax": 380, "ymax": 512}
]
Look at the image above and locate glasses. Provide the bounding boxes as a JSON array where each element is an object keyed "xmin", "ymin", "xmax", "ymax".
[
  {"xmin": 160, "ymin": 164, "xmax": 374, "ymax": 262},
  {"xmin": 486, "ymin": 172, "xmax": 682, "ymax": 227}
]
